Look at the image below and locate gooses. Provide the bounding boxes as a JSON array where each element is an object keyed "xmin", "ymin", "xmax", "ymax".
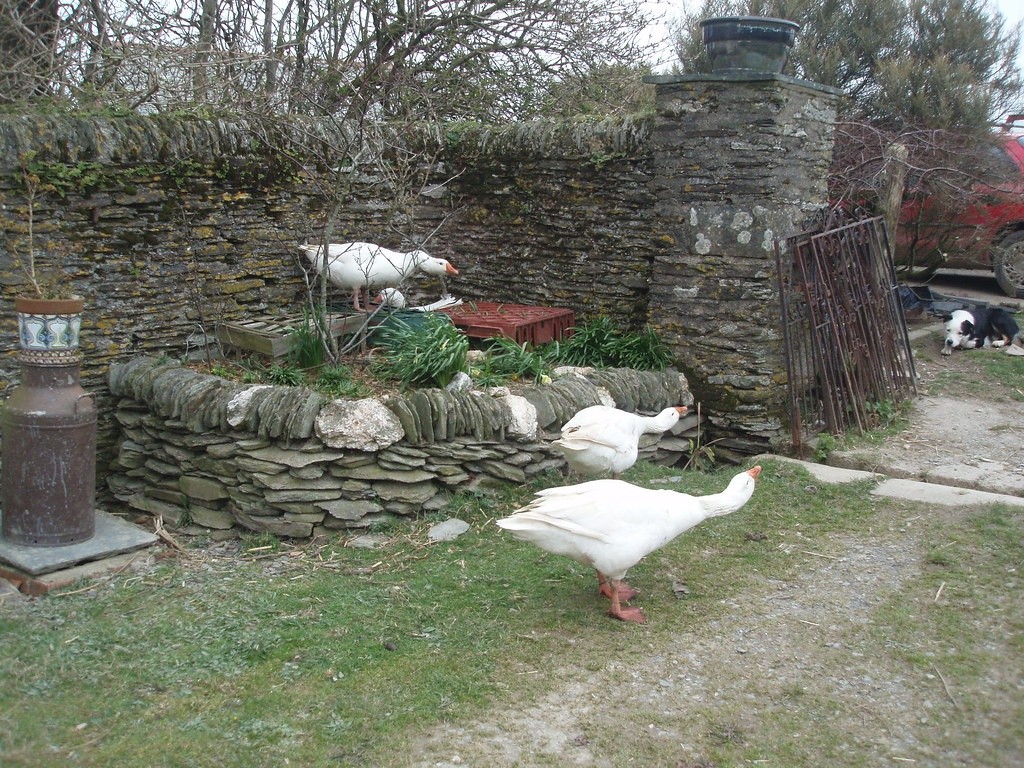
[
  {"xmin": 298, "ymin": 239, "xmax": 460, "ymax": 310},
  {"xmin": 495, "ymin": 465, "xmax": 763, "ymax": 624},
  {"xmin": 551, "ymin": 404, "xmax": 688, "ymax": 481}
]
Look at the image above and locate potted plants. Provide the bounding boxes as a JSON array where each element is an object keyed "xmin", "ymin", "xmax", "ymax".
[
  {"xmin": 0, "ymin": 148, "xmax": 86, "ymax": 349},
  {"xmin": 370, "ymin": 128, "xmax": 468, "ymax": 359}
]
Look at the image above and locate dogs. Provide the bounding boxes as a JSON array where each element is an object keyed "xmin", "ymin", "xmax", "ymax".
[{"xmin": 938, "ymin": 306, "xmax": 1024, "ymax": 356}]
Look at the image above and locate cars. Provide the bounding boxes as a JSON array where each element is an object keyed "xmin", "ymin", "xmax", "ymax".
[{"xmin": 828, "ymin": 117, "xmax": 1024, "ymax": 298}]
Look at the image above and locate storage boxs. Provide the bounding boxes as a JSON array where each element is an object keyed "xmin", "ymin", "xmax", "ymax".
[
  {"xmin": 216, "ymin": 311, "xmax": 369, "ymax": 367},
  {"xmin": 438, "ymin": 299, "xmax": 576, "ymax": 352}
]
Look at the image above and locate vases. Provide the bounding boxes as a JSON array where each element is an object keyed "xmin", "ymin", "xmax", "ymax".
[{"xmin": 699, "ymin": 15, "xmax": 801, "ymax": 74}]
